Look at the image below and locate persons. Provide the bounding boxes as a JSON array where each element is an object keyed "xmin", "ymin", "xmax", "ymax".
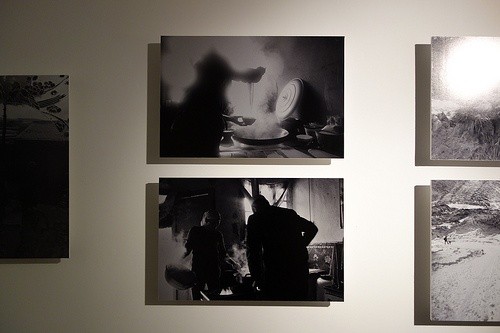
[
  {"xmin": 174, "ymin": 207, "xmax": 243, "ymax": 301},
  {"xmin": 167, "ymin": 46, "xmax": 266, "ymax": 156},
  {"xmin": 245, "ymin": 194, "xmax": 319, "ymax": 300}
]
